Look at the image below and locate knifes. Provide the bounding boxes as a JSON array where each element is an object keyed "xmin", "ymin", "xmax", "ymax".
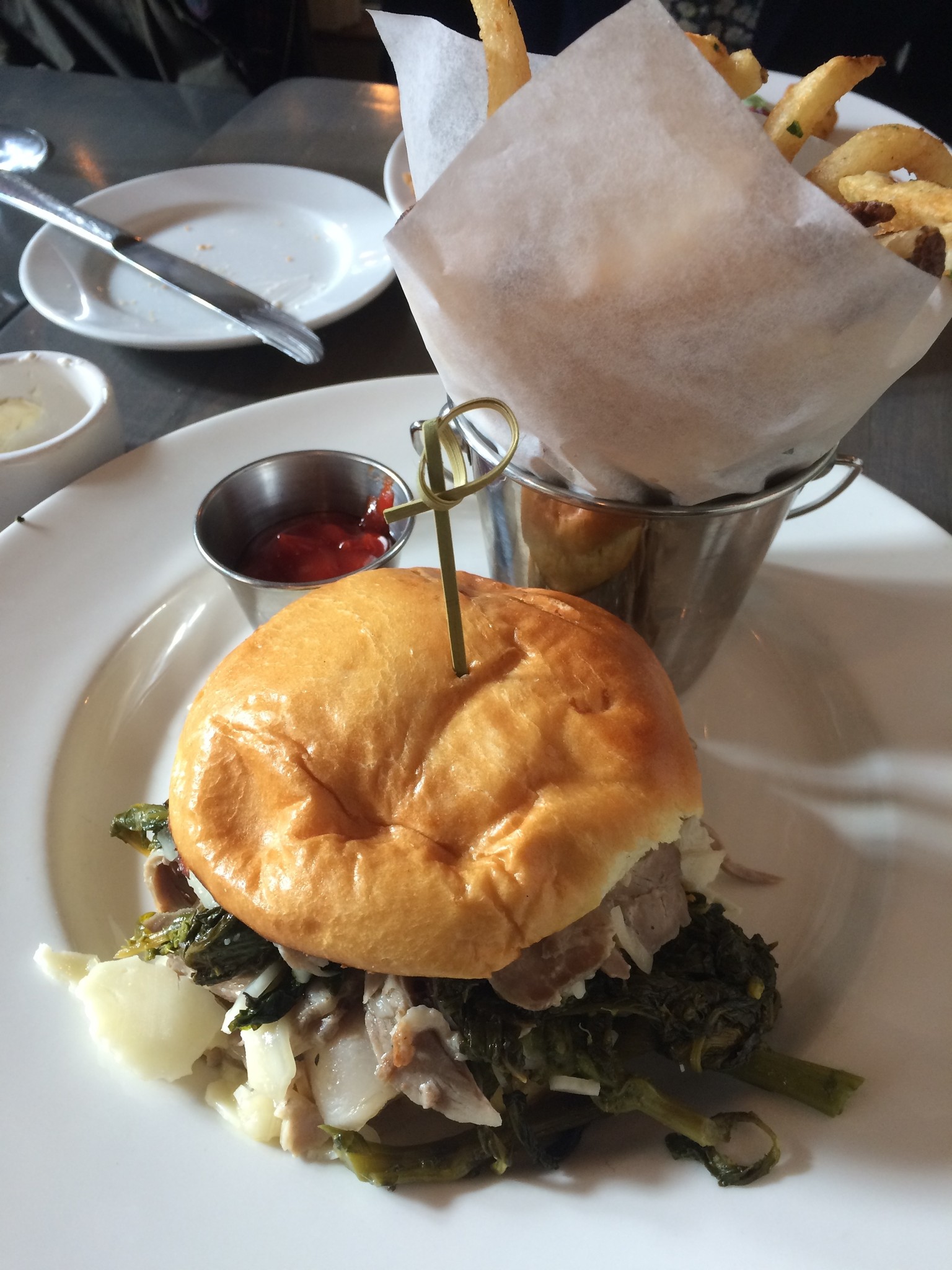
[{"xmin": 1, "ymin": 170, "xmax": 325, "ymax": 366}]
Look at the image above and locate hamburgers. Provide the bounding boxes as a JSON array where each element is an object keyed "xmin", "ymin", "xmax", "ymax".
[{"xmin": 30, "ymin": 567, "xmax": 867, "ymax": 1189}]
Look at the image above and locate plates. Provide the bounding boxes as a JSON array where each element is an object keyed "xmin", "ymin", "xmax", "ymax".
[
  {"xmin": 1, "ymin": 375, "xmax": 952, "ymax": 1270},
  {"xmin": 384, "ymin": 68, "xmax": 952, "ymax": 242},
  {"xmin": 0, "ymin": 165, "xmax": 394, "ymax": 349}
]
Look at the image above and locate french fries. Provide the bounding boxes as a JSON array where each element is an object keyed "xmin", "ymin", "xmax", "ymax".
[{"xmin": 472, "ymin": 0, "xmax": 950, "ymax": 282}]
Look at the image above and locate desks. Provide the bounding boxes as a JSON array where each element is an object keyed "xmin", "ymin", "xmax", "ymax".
[{"xmin": 0, "ymin": 60, "xmax": 952, "ymax": 540}]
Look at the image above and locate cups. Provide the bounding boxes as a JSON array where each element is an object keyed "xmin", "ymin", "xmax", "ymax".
[
  {"xmin": 0, "ymin": 350, "xmax": 118, "ymax": 527},
  {"xmin": 408, "ymin": 397, "xmax": 863, "ymax": 690},
  {"xmin": 193, "ymin": 451, "xmax": 415, "ymax": 630}
]
